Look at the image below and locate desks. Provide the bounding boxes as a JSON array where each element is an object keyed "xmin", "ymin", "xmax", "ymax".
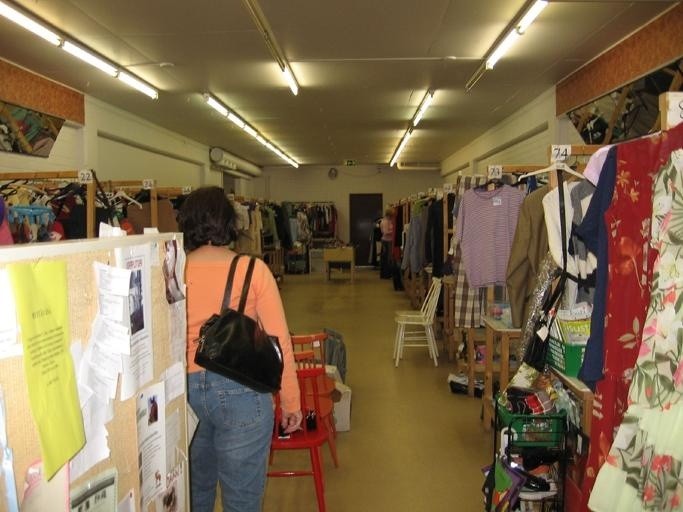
[{"xmin": 324, "ymin": 245, "xmax": 354, "ymax": 285}]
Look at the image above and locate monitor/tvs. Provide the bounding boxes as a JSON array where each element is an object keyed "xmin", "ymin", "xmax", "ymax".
[{"xmin": 543, "ymin": 300, "xmax": 592, "ymax": 369}]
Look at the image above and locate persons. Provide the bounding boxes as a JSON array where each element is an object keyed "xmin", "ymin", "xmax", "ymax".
[
  {"xmin": 172, "ymin": 184, "xmax": 303, "ymax": 511},
  {"xmin": 379, "ymin": 205, "xmax": 396, "ymax": 280}
]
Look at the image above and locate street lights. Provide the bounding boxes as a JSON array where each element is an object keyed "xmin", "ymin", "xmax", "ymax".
[{"xmin": 193, "ymin": 251, "xmax": 284, "ymax": 393}]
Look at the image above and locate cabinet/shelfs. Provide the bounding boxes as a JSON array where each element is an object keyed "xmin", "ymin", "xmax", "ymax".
[
  {"xmin": 0, "ymin": 174, "xmax": 336, "ymax": 511},
  {"xmin": 383, "ymin": 92, "xmax": 683, "ymax": 512}
]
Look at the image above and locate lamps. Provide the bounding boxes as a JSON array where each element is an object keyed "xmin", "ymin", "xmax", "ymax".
[
  {"xmin": 202, "ymin": 92, "xmax": 300, "ymax": 167},
  {"xmin": 481, "ymin": 3, "xmax": 547, "ymax": 71},
  {"xmin": 390, "ymin": 89, "xmax": 435, "ymax": 167},
  {"xmin": 245, "ymin": 2, "xmax": 300, "ymax": 95},
  {"xmin": 2, "ymin": 0, "xmax": 159, "ymax": 100}
]
